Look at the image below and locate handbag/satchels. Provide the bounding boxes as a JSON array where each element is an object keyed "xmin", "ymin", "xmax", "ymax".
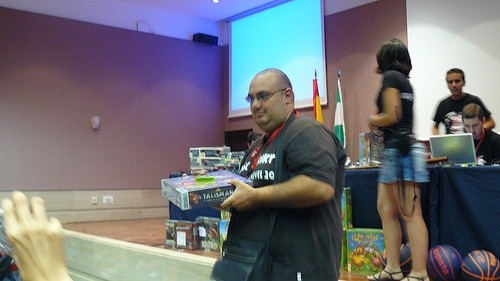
[{"xmin": 210, "ymin": 239, "xmax": 271, "ymax": 281}]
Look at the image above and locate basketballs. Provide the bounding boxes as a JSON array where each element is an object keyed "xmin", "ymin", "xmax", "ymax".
[{"xmin": 382, "ymin": 243, "xmax": 500, "ymax": 281}]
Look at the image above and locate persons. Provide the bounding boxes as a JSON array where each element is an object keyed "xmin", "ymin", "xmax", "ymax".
[
  {"xmin": 211, "ymin": 68, "xmax": 346, "ymax": 281},
  {"xmin": 462, "ymin": 103, "xmax": 500, "ymax": 165},
  {"xmin": 432, "ymin": 68, "xmax": 495, "ymax": 135},
  {"xmin": 247, "ymin": 132, "xmax": 261, "ymax": 147},
  {"xmin": 368, "ymin": 37, "xmax": 429, "ymax": 281},
  {"xmin": 1, "ymin": 191, "xmax": 73, "ymax": 281}
]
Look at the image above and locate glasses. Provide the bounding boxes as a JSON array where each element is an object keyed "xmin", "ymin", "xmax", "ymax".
[{"xmin": 245, "ymin": 88, "xmax": 286, "ymax": 102}]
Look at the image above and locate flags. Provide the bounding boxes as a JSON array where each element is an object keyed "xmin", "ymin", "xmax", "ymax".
[
  {"xmin": 313, "ymin": 79, "xmax": 323, "ymax": 124},
  {"xmin": 334, "ymin": 80, "xmax": 346, "ymax": 149}
]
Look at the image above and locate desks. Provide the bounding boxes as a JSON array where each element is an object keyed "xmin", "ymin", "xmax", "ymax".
[{"xmin": 169, "ymin": 164, "xmax": 500, "ymax": 263}]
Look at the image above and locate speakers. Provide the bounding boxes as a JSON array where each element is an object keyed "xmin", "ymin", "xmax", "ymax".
[{"xmin": 193, "ymin": 33, "xmax": 219, "ymax": 46}]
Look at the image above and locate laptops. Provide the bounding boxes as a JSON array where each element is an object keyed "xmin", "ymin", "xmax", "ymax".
[{"xmin": 429, "ymin": 133, "xmax": 482, "ymax": 167}]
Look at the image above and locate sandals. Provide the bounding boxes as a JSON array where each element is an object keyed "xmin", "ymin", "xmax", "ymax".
[
  {"xmin": 400, "ymin": 274, "xmax": 430, "ymax": 280},
  {"xmin": 366, "ymin": 269, "xmax": 404, "ymax": 281}
]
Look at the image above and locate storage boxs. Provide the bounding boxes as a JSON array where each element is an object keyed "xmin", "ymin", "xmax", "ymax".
[
  {"xmin": 190, "ymin": 147, "xmax": 246, "ymax": 175},
  {"xmin": 340, "ymin": 186, "xmax": 386, "ymax": 274},
  {"xmin": 358, "ymin": 132, "xmax": 385, "ymax": 167},
  {"xmin": 165, "ymin": 217, "xmax": 231, "ymax": 256},
  {"xmin": 161, "ymin": 170, "xmax": 254, "ymax": 211}
]
